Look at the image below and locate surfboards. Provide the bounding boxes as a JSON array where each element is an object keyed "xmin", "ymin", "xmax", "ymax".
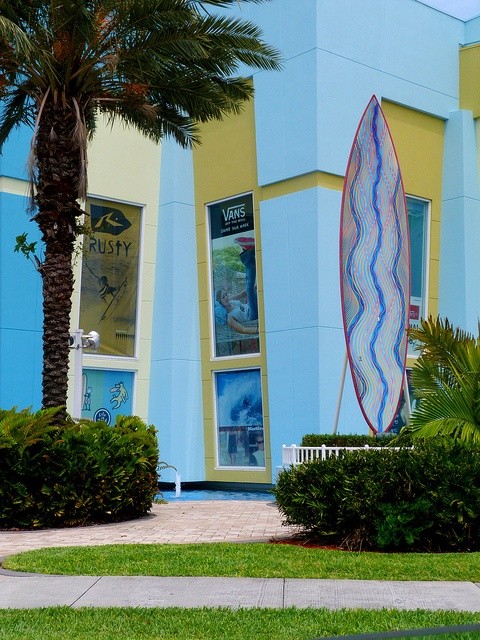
[{"xmin": 336, "ymin": 92, "xmax": 413, "ymax": 437}]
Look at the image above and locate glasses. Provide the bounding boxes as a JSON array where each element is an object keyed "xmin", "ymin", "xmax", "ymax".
[{"xmin": 257, "ymin": 440, "xmax": 264, "ymax": 445}]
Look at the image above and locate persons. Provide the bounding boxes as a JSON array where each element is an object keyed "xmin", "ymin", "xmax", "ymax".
[
  {"xmin": 225, "ymin": 431, "xmax": 238, "ymax": 464},
  {"xmin": 215, "ymin": 237, "xmax": 259, "ymax": 334},
  {"xmin": 86, "ymin": 263, "xmax": 122, "ymax": 307},
  {"xmin": 252, "ymin": 434, "xmax": 265, "ymax": 466}
]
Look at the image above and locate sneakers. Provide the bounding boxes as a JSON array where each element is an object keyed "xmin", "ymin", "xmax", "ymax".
[{"xmin": 234, "ymin": 237, "xmax": 255, "ymax": 250}]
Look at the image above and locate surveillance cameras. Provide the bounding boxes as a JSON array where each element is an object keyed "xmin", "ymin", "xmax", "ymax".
[{"xmin": 86, "ymin": 330, "xmax": 100, "ymax": 348}]
